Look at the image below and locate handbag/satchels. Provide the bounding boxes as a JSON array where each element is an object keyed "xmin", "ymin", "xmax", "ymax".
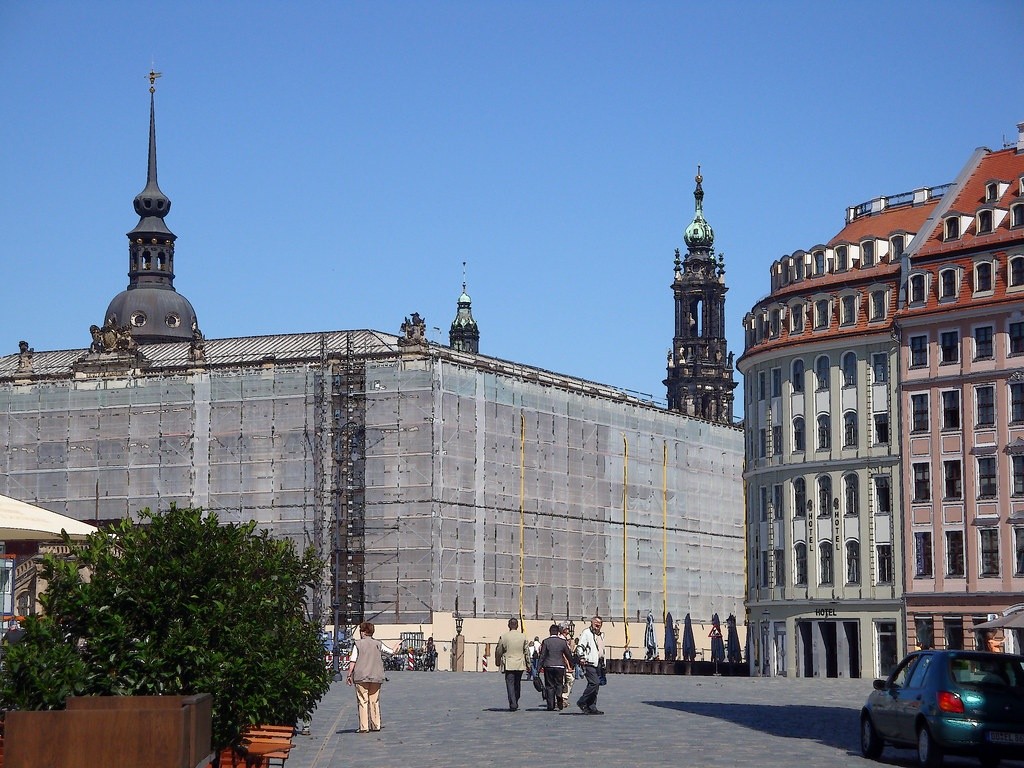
[
  {"xmin": 598, "ymin": 661, "xmax": 607, "ymax": 686},
  {"xmin": 533, "ymin": 677, "xmax": 544, "ymax": 692}
]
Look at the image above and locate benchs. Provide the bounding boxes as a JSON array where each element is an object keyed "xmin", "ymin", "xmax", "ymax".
[{"xmin": 206, "ymin": 725, "xmax": 295, "ymax": 768}]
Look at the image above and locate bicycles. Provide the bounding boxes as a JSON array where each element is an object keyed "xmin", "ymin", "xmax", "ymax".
[{"xmin": 423, "ymin": 651, "xmax": 438, "ymax": 671}]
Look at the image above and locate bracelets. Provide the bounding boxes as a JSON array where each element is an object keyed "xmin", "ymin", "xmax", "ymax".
[{"xmin": 347, "ymin": 675, "xmax": 351, "ymax": 678}]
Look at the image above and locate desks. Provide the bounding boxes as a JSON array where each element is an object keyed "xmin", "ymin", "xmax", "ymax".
[{"xmin": 238, "ymin": 743, "xmax": 295, "ymax": 768}]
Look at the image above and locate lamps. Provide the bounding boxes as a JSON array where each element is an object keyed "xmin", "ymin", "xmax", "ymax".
[
  {"xmin": 569, "ymin": 620, "xmax": 575, "ymax": 639},
  {"xmin": 455, "ymin": 616, "xmax": 463, "ymax": 635},
  {"xmin": 674, "ymin": 625, "xmax": 679, "ymax": 636}
]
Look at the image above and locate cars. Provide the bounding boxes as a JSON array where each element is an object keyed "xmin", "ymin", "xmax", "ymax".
[{"xmin": 859, "ymin": 648, "xmax": 1024, "ymax": 768}]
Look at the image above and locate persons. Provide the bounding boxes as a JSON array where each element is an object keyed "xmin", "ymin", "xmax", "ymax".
[
  {"xmin": 494, "ymin": 616, "xmax": 607, "ymax": 715},
  {"xmin": 424, "ymin": 637, "xmax": 435, "ymax": 667},
  {"xmin": 915, "ymin": 641, "xmax": 923, "ymax": 651},
  {"xmin": 301, "ymin": 692, "xmax": 312, "ymax": 735},
  {"xmin": 985, "ymin": 632, "xmax": 1007, "ymax": 652},
  {"xmin": 345, "ymin": 621, "xmax": 404, "ymax": 734}
]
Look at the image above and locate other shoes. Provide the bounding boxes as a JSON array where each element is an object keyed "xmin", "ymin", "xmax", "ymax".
[
  {"xmin": 590, "ymin": 710, "xmax": 605, "ymax": 716},
  {"xmin": 355, "ymin": 729, "xmax": 369, "ymax": 733},
  {"xmin": 577, "ymin": 701, "xmax": 589, "ymax": 715},
  {"xmin": 512, "ymin": 707, "xmax": 518, "ymax": 711},
  {"xmin": 557, "ymin": 696, "xmax": 564, "ymax": 711}
]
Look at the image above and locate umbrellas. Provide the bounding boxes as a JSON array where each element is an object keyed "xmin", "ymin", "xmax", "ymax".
[
  {"xmin": 643, "ymin": 611, "xmax": 741, "ymax": 673},
  {"xmin": 972, "ymin": 611, "xmax": 1024, "ymax": 631}
]
